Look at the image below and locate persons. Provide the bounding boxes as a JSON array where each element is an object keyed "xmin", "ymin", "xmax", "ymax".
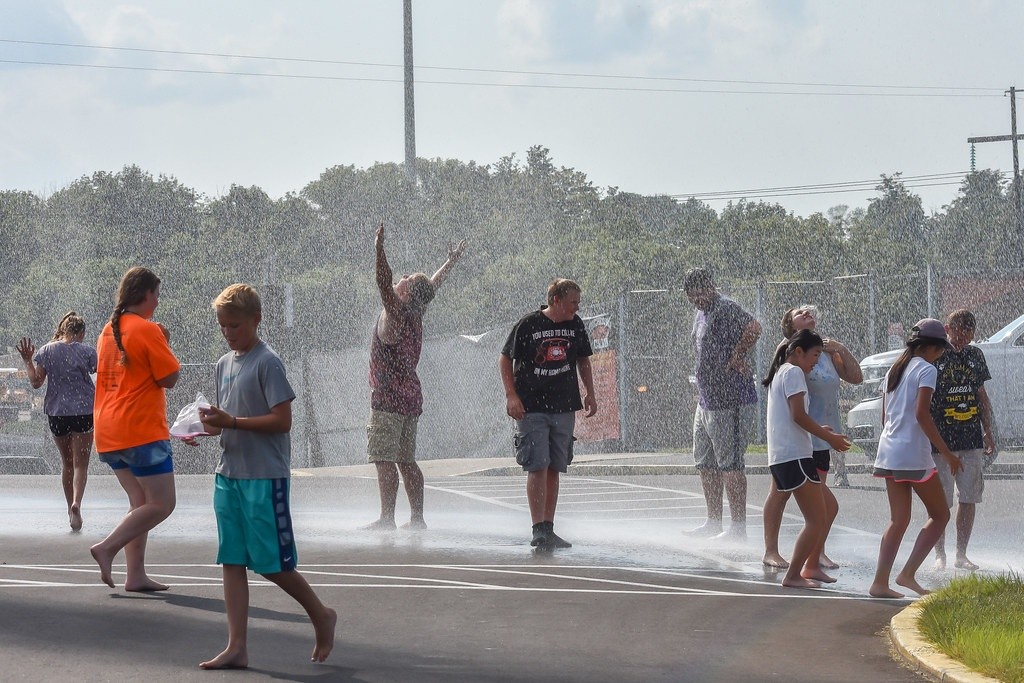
[
  {"xmin": 927, "ymin": 309, "xmax": 995, "ymax": 571},
  {"xmin": 766, "ymin": 307, "xmax": 863, "ymax": 569},
  {"xmin": 498, "ymin": 278, "xmax": 596, "ymax": 548},
  {"xmin": 681, "ymin": 267, "xmax": 762, "ymax": 541},
  {"xmin": 761, "ymin": 330, "xmax": 850, "ymax": 588},
  {"xmin": 89, "ymin": 266, "xmax": 180, "ymax": 591},
  {"xmin": 358, "ymin": 223, "xmax": 467, "ymax": 530},
  {"xmin": 868, "ymin": 317, "xmax": 966, "ymax": 599},
  {"xmin": 181, "ymin": 283, "xmax": 338, "ymax": 670},
  {"xmin": 16, "ymin": 312, "xmax": 98, "ymax": 530}
]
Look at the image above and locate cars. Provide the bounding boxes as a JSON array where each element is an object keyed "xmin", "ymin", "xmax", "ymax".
[
  {"xmin": 846, "ymin": 313, "xmax": 1024, "ymax": 466},
  {"xmin": 0, "ymin": 454, "xmax": 56, "ymax": 476}
]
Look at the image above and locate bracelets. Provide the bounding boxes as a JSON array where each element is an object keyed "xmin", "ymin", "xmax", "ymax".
[{"xmin": 233, "ymin": 416, "xmax": 237, "ymax": 428}]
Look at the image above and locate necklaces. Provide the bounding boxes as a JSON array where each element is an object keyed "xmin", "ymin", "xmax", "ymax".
[{"xmin": 224, "ymin": 339, "xmax": 260, "ymax": 408}]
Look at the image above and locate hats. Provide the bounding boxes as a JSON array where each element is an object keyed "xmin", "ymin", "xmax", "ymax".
[{"xmin": 912, "ymin": 318, "xmax": 957, "ymax": 351}]
